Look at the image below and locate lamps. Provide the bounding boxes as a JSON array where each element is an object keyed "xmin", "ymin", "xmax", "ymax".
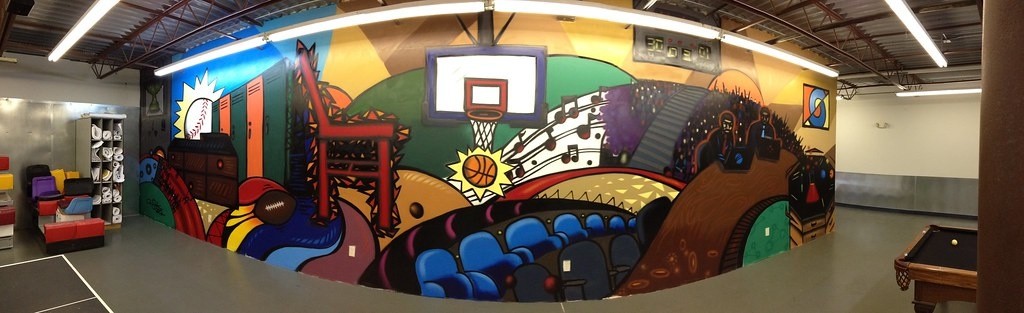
[
  {"xmin": 48, "ymin": 0, "xmax": 120, "ymax": 62},
  {"xmin": 154, "ymin": 0, "xmax": 839, "ymax": 77},
  {"xmin": 884, "ymin": 0, "xmax": 948, "ymax": 68}
]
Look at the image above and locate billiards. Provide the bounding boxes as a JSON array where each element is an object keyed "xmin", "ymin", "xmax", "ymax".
[
  {"xmin": 898, "ymin": 276, "xmax": 907, "ymax": 287},
  {"xmin": 952, "ymin": 239, "xmax": 958, "ymax": 246}
]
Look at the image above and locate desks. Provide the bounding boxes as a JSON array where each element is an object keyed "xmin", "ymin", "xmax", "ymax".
[{"xmin": 894, "ymin": 224, "xmax": 977, "ymax": 313}]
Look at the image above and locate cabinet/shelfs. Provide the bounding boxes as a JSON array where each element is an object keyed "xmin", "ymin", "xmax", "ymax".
[{"xmin": 75, "ymin": 117, "xmax": 124, "ymax": 229}]
